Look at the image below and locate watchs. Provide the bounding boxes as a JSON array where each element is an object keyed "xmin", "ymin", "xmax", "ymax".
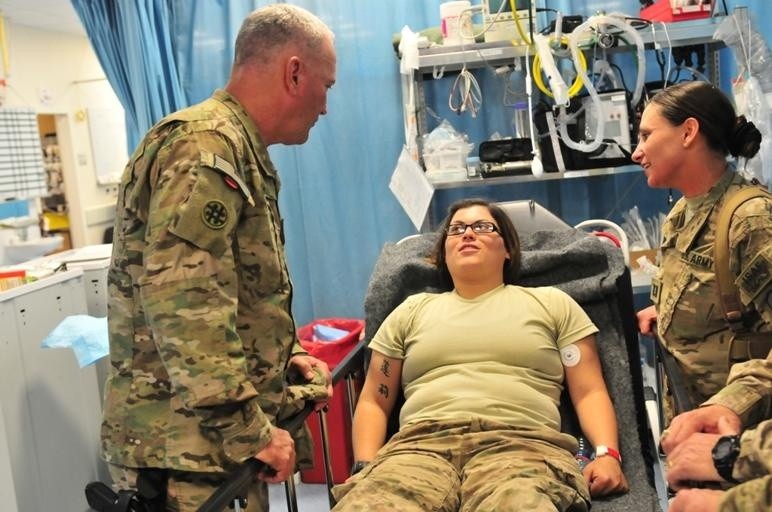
[
  {"xmin": 590, "ymin": 445, "xmax": 624, "ymax": 465},
  {"xmin": 711, "ymin": 434, "xmax": 740, "ymax": 486}
]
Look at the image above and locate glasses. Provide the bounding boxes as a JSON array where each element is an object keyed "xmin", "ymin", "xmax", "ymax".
[{"xmin": 445, "ymin": 222, "xmax": 502, "ymax": 236}]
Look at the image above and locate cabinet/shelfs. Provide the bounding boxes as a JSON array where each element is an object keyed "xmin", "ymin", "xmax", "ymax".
[{"xmin": 398, "ymin": 4, "xmax": 748, "ymax": 297}]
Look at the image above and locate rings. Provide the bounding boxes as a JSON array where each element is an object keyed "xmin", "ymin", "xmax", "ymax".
[{"xmin": 666, "ymin": 460, "xmax": 676, "ymax": 467}]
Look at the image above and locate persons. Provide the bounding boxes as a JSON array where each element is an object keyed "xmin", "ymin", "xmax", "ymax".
[
  {"xmin": 83, "ymin": 0, "xmax": 340, "ymax": 510},
  {"xmin": 665, "ymin": 415, "xmax": 772, "ymax": 511},
  {"xmin": 328, "ymin": 196, "xmax": 632, "ymax": 511},
  {"xmin": 629, "ymin": 79, "xmax": 772, "ymax": 453}
]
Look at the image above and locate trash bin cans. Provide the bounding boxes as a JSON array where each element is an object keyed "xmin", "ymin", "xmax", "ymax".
[{"xmin": 296, "ymin": 318, "xmax": 365, "ymax": 484}]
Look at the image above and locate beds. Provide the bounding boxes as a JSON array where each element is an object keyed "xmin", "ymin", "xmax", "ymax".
[{"xmin": 195, "ymin": 201, "xmax": 670, "ymax": 511}]
